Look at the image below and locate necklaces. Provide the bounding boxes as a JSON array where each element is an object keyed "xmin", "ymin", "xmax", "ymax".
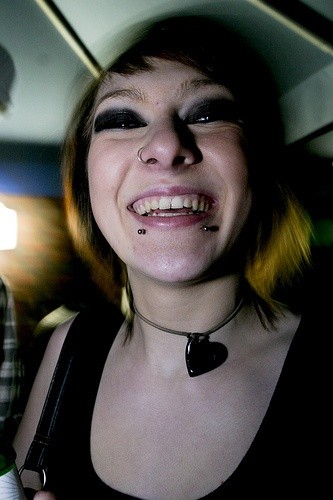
[{"xmin": 126, "ymin": 291, "xmax": 247, "ymax": 377}]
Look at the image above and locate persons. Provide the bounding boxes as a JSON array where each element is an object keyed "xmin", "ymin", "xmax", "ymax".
[
  {"xmin": 7, "ymin": 19, "xmax": 332, "ymax": 500},
  {"xmin": 0, "ymin": 48, "xmax": 22, "ymax": 447}
]
